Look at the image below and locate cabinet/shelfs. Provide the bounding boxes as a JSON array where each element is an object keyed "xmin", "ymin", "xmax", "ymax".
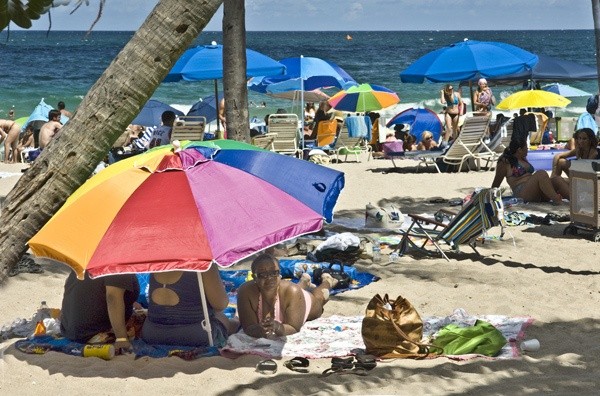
[{"xmin": 567, "ymin": 167, "xmax": 600, "ymax": 241}]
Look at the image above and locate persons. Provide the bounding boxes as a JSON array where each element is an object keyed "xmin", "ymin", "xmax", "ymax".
[
  {"xmin": 556, "ymin": 131, "xmax": 579, "ymax": 165},
  {"xmin": 519, "ymin": 109, "xmax": 526, "ymax": 115},
  {"xmin": 123, "ymin": 128, "xmax": 137, "ymax": 145},
  {"xmin": 219, "ymin": 96, "xmax": 228, "ymax": 131},
  {"xmin": 58, "ymin": 102, "xmax": 72, "ymax": 116},
  {"xmin": 258, "ymin": 101, "xmax": 266, "ymax": 107},
  {"xmin": 577, "ymin": 98, "xmax": 598, "ymax": 135},
  {"xmin": 61, "ymin": 269, "xmax": 140, "ymax": 351},
  {"xmin": 346, "ymin": 114, "xmax": 351, "ymax": 116},
  {"xmin": 474, "ymin": 77, "xmax": 496, "ymax": 111},
  {"xmin": 116, "ymin": 336, "xmax": 130, "ymax": 342},
  {"xmin": 144, "ymin": 265, "xmax": 238, "ymax": 348},
  {"xmin": 366, "ymin": 112, "xmax": 378, "ymax": 151},
  {"xmin": 252, "ymin": 115, "xmax": 260, "ymax": 122},
  {"xmin": 145, "ymin": 110, "xmax": 175, "ymax": 151},
  {"xmin": 554, "ymin": 128, "xmax": 599, "ymax": 176},
  {"xmin": 592, "ymin": 137, "xmax": 598, "ymax": 146},
  {"xmin": 491, "ymin": 135, "xmax": 570, "ymax": 204},
  {"xmin": 304, "ymin": 103, "xmax": 316, "ymax": 117},
  {"xmin": 513, "ymin": 113, "xmax": 519, "ymax": 118},
  {"xmin": 250, "ymin": 100, "xmax": 257, "ymax": 107},
  {"xmin": 39, "ymin": 109, "xmax": 63, "ymax": 149},
  {"xmin": 355, "ymin": 112, "xmax": 360, "ymax": 115},
  {"xmin": 411, "ymin": 141, "xmax": 417, "ymax": 153},
  {"xmin": 394, "ymin": 125, "xmax": 407, "ymax": 141},
  {"xmin": 0, "ymin": 119, "xmax": 20, "ymax": 162},
  {"xmin": 263, "ymin": 114, "xmax": 271, "ymax": 125},
  {"xmin": 276, "ymin": 108, "xmax": 288, "ymax": 118},
  {"xmin": 439, "ymin": 84, "xmax": 464, "ymax": 143},
  {"xmin": 8, "ymin": 104, "xmax": 16, "ymax": 117},
  {"xmin": 18, "ymin": 125, "xmax": 35, "ymax": 163},
  {"xmin": 417, "ymin": 131, "xmax": 437, "ymax": 151},
  {"xmin": 315, "ymin": 100, "xmax": 332, "ymax": 123},
  {"xmin": 237, "ymin": 256, "xmax": 339, "ymax": 337}
]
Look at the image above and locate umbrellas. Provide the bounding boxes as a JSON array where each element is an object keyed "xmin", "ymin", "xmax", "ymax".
[
  {"xmin": 498, "ymin": 92, "xmax": 573, "ymax": 115},
  {"xmin": 131, "ymin": 100, "xmax": 184, "ymax": 127},
  {"xmin": 398, "ymin": 38, "xmax": 541, "ymax": 84},
  {"xmin": 21, "ymin": 97, "xmax": 70, "ymax": 130},
  {"xmin": 186, "ymin": 91, "xmax": 225, "ymax": 133},
  {"xmin": 327, "ymin": 83, "xmax": 402, "ymax": 116},
  {"xmin": 460, "ymin": 54, "xmax": 599, "ymax": 83},
  {"xmin": 270, "ymin": 89, "xmax": 332, "ymax": 101},
  {"xmin": 541, "ymin": 82, "xmax": 593, "ymax": 97},
  {"xmin": 161, "ymin": 40, "xmax": 287, "ymax": 82},
  {"xmin": 22, "ymin": 138, "xmax": 346, "ymax": 347}
]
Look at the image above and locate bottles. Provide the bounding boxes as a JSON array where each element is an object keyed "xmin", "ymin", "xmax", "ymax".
[
  {"xmin": 81, "ymin": 345, "xmax": 115, "ymax": 360},
  {"xmin": 390, "ymin": 252, "xmax": 398, "ymax": 261},
  {"xmin": 372, "ymin": 242, "xmax": 381, "ymax": 262},
  {"xmin": 41, "ymin": 301, "xmax": 48, "ymax": 309}
]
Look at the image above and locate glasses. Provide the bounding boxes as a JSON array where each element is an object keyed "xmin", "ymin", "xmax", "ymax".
[
  {"xmin": 254, "ymin": 269, "xmax": 280, "ymax": 279},
  {"xmin": 426, "ymin": 136, "xmax": 433, "ymax": 140},
  {"xmin": 449, "ymin": 87, "xmax": 454, "ymax": 91}
]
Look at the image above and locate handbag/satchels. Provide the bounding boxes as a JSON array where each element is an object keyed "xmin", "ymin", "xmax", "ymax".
[
  {"xmin": 315, "ymin": 258, "xmax": 350, "ymax": 290},
  {"xmin": 365, "ymin": 203, "xmax": 404, "ymax": 228},
  {"xmin": 362, "ymin": 293, "xmax": 444, "ymax": 360}
]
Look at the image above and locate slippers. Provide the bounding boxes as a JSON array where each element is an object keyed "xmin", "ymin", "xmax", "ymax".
[
  {"xmin": 430, "ymin": 198, "xmax": 449, "ymax": 204},
  {"xmin": 283, "ymin": 357, "xmax": 311, "ymax": 373},
  {"xmin": 354, "ymin": 354, "xmax": 376, "ymax": 370},
  {"xmin": 449, "ymin": 199, "xmax": 463, "ymax": 206},
  {"xmin": 256, "ymin": 359, "xmax": 278, "ymax": 375},
  {"xmin": 321, "ymin": 355, "xmax": 354, "ymax": 377}
]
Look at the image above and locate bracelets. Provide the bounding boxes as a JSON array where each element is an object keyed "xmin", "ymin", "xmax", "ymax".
[{"xmin": 276, "ymin": 323, "xmax": 281, "ymax": 334}]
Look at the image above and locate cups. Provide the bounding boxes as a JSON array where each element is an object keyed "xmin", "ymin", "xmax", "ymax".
[{"xmin": 520, "ymin": 339, "xmax": 540, "ymax": 351}]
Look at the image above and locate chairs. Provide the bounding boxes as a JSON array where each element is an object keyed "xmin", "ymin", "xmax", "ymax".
[
  {"xmin": 300, "ymin": 119, "xmax": 338, "ymax": 148},
  {"xmin": 253, "ymin": 133, "xmax": 279, "ymax": 154},
  {"xmin": 170, "ymin": 115, "xmax": 207, "ymax": 145},
  {"xmin": 404, "ymin": 110, "xmax": 495, "ymax": 173},
  {"xmin": 522, "ymin": 111, "xmax": 550, "ymax": 145},
  {"xmin": 392, "ymin": 185, "xmax": 507, "ymax": 262},
  {"xmin": 337, "ymin": 115, "xmax": 373, "ymax": 164},
  {"xmin": 473, "ymin": 117, "xmax": 516, "ymax": 172},
  {"xmin": 268, "ymin": 113, "xmax": 298, "ymax": 158}
]
